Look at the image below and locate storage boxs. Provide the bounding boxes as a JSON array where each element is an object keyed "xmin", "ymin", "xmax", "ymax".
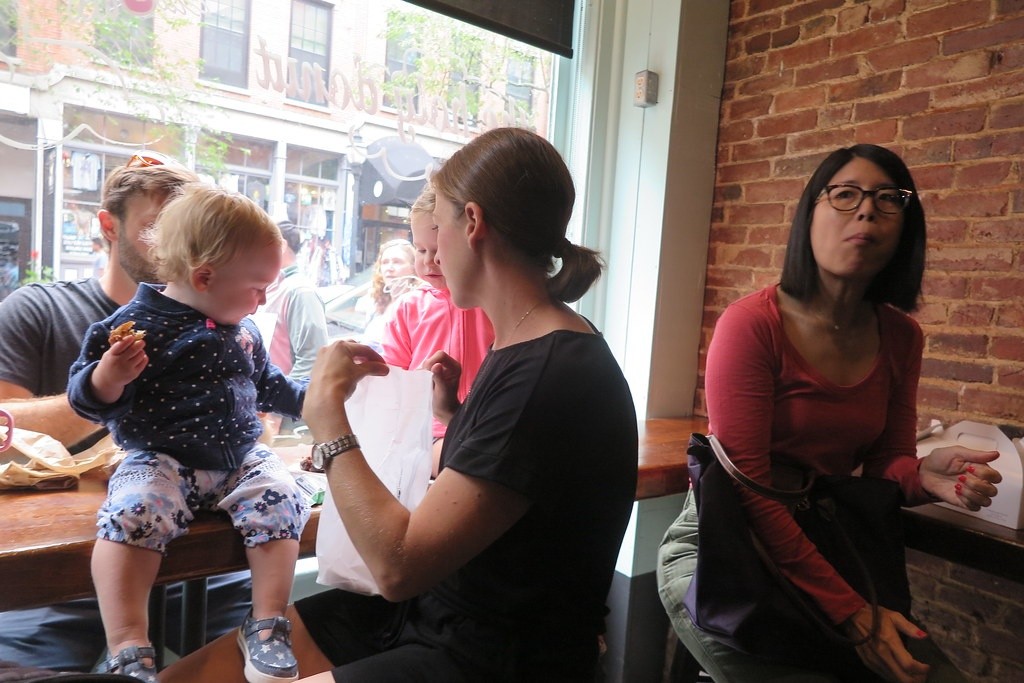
[{"xmin": 915, "ymin": 418, "xmax": 1024, "ymax": 529}]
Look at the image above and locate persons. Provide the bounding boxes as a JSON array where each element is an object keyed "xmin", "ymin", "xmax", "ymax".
[
  {"xmin": 0, "ymin": 124, "xmax": 639, "ymax": 683},
  {"xmin": 655, "ymin": 142, "xmax": 1002, "ymax": 683}
]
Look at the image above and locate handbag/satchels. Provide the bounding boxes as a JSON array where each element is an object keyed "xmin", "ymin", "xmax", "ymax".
[{"xmin": 685, "ymin": 431, "xmax": 912, "ymax": 663}]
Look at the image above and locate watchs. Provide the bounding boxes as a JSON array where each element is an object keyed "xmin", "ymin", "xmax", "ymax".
[{"xmin": 312, "ymin": 434, "xmax": 359, "ymax": 470}]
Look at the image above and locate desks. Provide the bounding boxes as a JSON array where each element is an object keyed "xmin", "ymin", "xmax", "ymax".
[{"xmin": 0, "ymin": 415, "xmax": 708, "ymax": 659}]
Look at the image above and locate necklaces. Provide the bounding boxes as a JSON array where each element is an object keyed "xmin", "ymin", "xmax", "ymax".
[{"xmin": 463, "ymin": 299, "xmax": 544, "ymax": 410}]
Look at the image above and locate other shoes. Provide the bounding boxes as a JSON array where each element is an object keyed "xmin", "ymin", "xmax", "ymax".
[
  {"xmin": 235, "ymin": 609, "xmax": 299, "ymax": 683},
  {"xmin": 96, "ymin": 642, "xmax": 156, "ymax": 683}
]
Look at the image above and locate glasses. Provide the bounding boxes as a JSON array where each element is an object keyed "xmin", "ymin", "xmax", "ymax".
[
  {"xmin": 126, "ymin": 150, "xmax": 183, "ymax": 167},
  {"xmin": 809, "ymin": 184, "xmax": 912, "ymax": 214}
]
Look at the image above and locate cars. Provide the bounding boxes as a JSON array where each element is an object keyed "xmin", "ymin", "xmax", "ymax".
[{"xmin": 316, "ymin": 257, "xmax": 384, "ymax": 340}]
